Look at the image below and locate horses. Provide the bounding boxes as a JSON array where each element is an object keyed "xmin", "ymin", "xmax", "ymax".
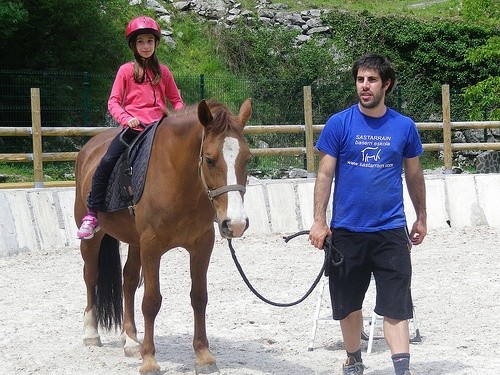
[{"xmin": 74, "ymin": 96, "xmax": 253, "ymax": 375}]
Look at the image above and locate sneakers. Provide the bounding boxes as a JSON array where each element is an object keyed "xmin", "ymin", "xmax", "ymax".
[
  {"xmin": 396, "ymin": 370, "xmax": 412, "ymax": 375},
  {"xmin": 76, "ymin": 215, "xmax": 100, "ymax": 239},
  {"xmin": 343, "ymin": 356, "xmax": 369, "ymax": 375}
]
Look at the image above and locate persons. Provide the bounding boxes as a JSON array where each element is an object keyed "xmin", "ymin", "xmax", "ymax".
[
  {"xmin": 309, "ymin": 52, "xmax": 428, "ymax": 375},
  {"xmin": 76, "ymin": 16, "xmax": 185, "ymax": 240}
]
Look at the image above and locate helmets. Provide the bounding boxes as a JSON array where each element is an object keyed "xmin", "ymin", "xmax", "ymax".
[{"xmin": 126, "ymin": 16, "xmax": 161, "ymax": 41}]
